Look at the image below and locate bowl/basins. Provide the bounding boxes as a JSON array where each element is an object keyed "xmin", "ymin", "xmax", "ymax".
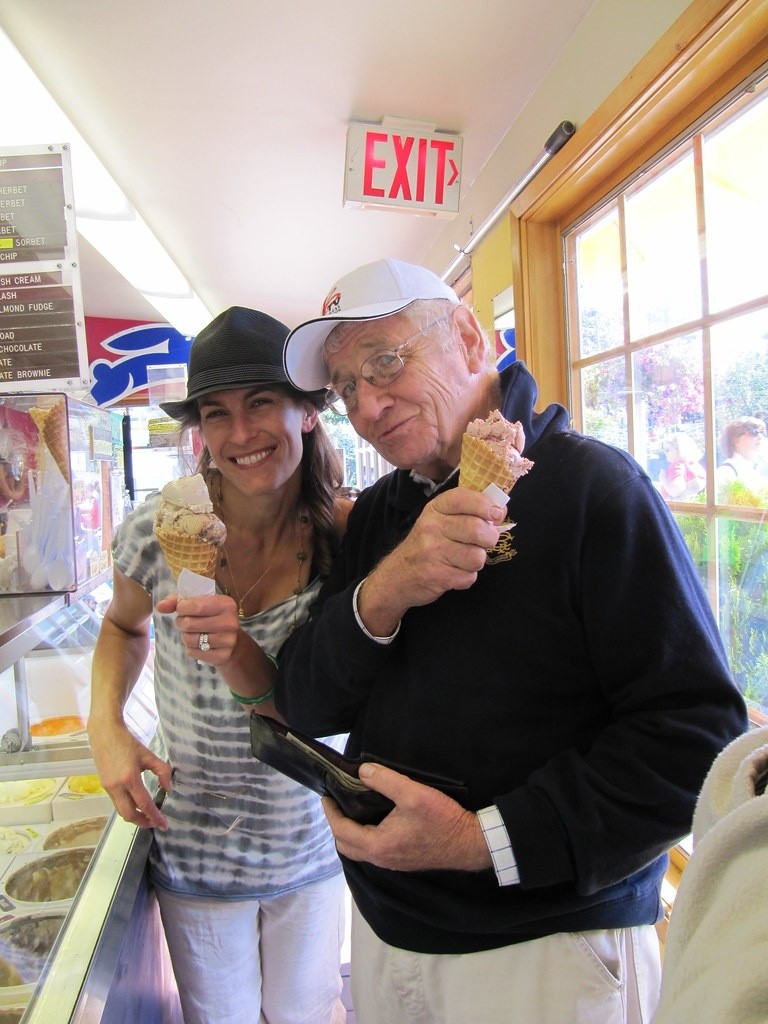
[
  {"xmin": 41, "ymin": 816, "xmax": 107, "ymax": 850},
  {"xmin": 4, "ymin": 849, "xmax": 124, "ymax": 906},
  {"xmin": 0, "ymin": 911, "xmax": 67, "ymax": 983},
  {"xmin": 30, "ymin": 716, "xmax": 83, "ymax": 736}
]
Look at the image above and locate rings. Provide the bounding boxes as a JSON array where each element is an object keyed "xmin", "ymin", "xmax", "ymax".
[{"xmin": 199, "ymin": 633, "xmax": 210, "ymax": 651}]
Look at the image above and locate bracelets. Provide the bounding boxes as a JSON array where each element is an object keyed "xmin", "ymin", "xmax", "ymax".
[{"xmin": 228, "ymin": 654, "xmax": 280, "ymax": 706}]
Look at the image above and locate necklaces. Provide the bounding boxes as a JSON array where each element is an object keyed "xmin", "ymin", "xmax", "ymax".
[
  {"xmin": 221, "ymin": 531, "xmax": 293, "ymax": 618},
  {"xmin": 209, "ymin": 469, "xmax": 309, "ymax": 633}
]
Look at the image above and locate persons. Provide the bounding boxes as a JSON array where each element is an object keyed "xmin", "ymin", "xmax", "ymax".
[
  {"xmin": 83, "ymin": 305, "xmax": 357, "ymax": 1024},
  {"xmin": 275, "ymin": 257, "xmax": 748, "ymax": 1023}
]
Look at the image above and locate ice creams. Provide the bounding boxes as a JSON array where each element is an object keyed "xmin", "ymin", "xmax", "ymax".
[
  {"xmin": 457, "ymin": 411, "xmax": 534, "ymax": 512},
  {"xmin": 154, "ymin": 472, "xmax": 228, "ymax": 600}
]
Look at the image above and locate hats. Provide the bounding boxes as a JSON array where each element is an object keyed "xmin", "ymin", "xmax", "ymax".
[
  {"xmin": 160, "ymin": 306, "xmax": 330, "ymax": 422},
  {"xmin": 281, "ymin": 256, "xmax": 462, "ymax": 390}
]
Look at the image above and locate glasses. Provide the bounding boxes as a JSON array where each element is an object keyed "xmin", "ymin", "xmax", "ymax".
[
  {"xmin": 325, "ymin": 315, "xmax": 453, "ymax": 415},
  {"xmin": 734, "ymin": 427, "xmax": 767, "ymax": 437}
]
